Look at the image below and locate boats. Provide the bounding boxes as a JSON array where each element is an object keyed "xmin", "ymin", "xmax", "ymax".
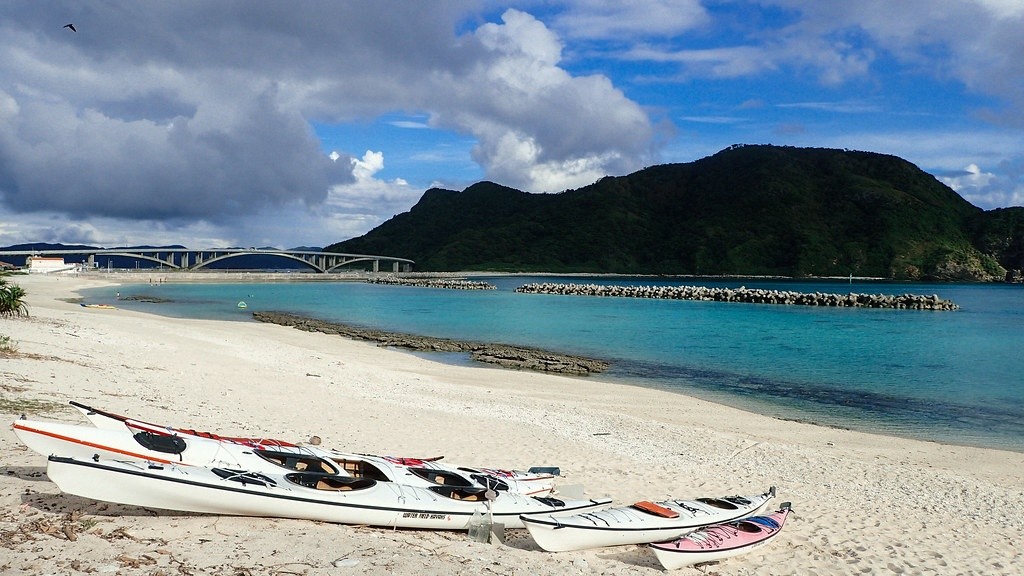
[
  {"xmin": 518, "ymin": 485, "xmax": 776, "ymax": 552},
  {"xmin": 9, "ymin": 397, "xmax": 613, "ymax": 528},
  {"xmin": 645, "ymin": 500, "xmax": 796, "ymax": 571}
]
